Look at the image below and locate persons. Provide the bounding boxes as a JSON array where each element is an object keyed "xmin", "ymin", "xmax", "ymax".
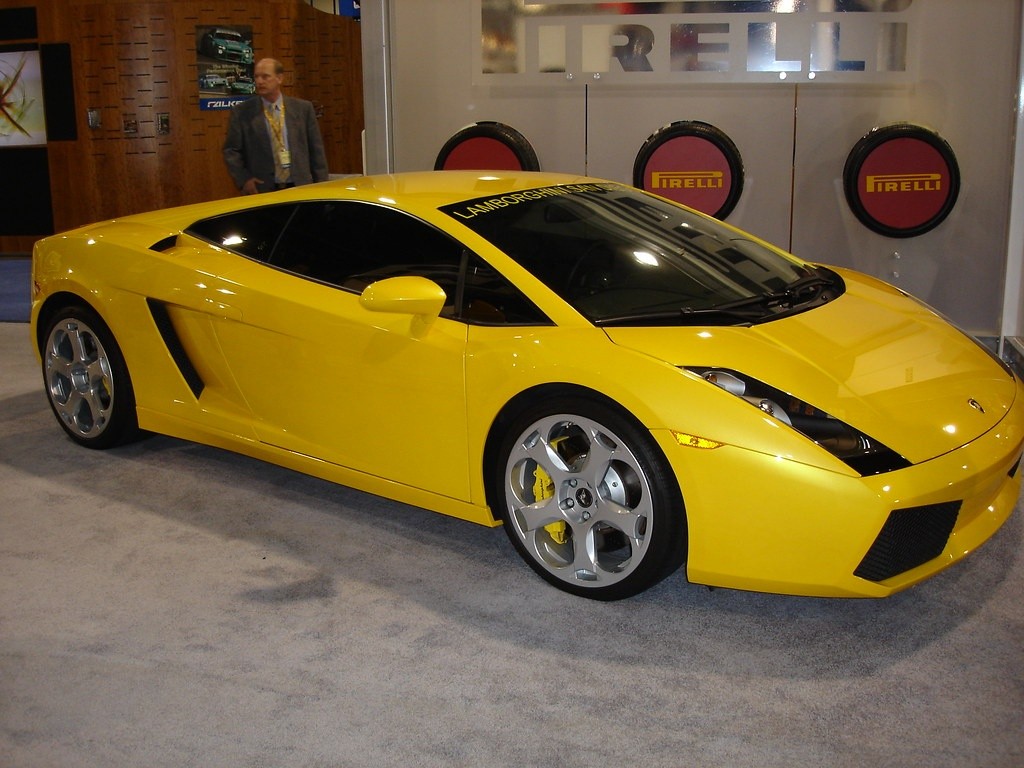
[{"xmin": 223, "ymin": 58, "xmax": 329, "ymax": 195}]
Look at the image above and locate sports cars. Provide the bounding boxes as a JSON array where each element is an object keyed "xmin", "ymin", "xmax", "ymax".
[{"xmin": 31, "ymin": 168, "xmax": 1022, "ymax": 602}]
